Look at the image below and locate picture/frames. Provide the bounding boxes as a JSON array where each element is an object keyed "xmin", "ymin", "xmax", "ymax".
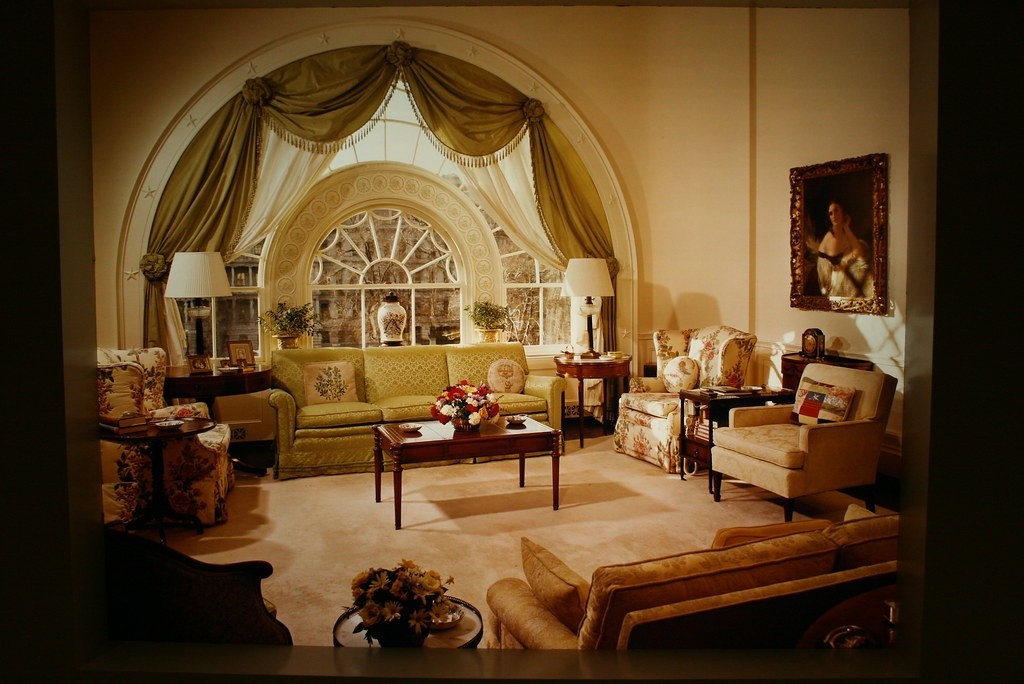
[
  {"xmin": 790, "ymin": 153, "xmax": 889, "ymax": 316},
  {"xmin": 186, "ymin": 355, "xmax": 213, "ymax": 375},
  {"xmin": 226, "ymin": 341, "xmax": 256, "ymax": 367}
]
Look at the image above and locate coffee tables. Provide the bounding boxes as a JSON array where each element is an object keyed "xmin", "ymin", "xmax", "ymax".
[{"xmin": 372, "ymin": 414, "xmax": 563, "ymax": 531}]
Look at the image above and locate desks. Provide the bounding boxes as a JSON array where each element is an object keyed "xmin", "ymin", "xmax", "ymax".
[
  {"xmin": 554, "ymin": 354, "xmax": 633, "ymax": 449},
  {"xmin": 99, "ymin": 417, "xmax": 217, "ymax": 546},
  {"xmin": 163, "ymin": 366, "xmax": 273, "ymax": 478}
]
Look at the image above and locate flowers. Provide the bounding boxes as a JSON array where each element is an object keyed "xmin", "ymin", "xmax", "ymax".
[
  {"xmin": 430, "ymin": 380, "xmax": 499, "ymax": 425},
  {"xmin": 341, "ymin": 558, "xmax": 455, "ymax": 648}
]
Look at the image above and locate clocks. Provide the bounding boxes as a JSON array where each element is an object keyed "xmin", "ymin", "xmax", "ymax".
[{"xmin": 801, "ymin": 328, "xmax": 825, "ymax": 359}]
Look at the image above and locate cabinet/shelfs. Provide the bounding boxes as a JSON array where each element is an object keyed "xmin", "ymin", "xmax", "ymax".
[
  {"xmin": 332, "ymin": 594, "xmax": 483, "ymax": 649},
  {"xmin": 781, "ymin": 352, "xmax": 875, "ymax": 391}
]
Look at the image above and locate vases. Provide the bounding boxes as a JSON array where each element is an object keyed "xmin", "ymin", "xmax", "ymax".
[
  {"xmin": 377, "ymin": 599, "xmax": 432, "ymax": 648},
  {"xmin": 452, "ymin": 415, "xmax": 481, "ymax": 432}
]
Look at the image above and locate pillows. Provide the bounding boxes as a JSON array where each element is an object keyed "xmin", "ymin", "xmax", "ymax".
[
  {"xmin": 488, "ymin": 359, "xmax": 525, "ymax": 393},
  {"xmin": 520, "ymin": 537, "xmax": 590, "ymax": 633},
  {"xmin": 304, "ymin": 362, "xmax": 359, "ymax": 406},
  {"xmin": 663, "ymin": 356, "xmax": 698, "ymax": 393},
  {"xmin": 789, "ymin": 376, "xmax": 855, "ymax": 426},
  {"xmin": 712, "ymin": 519, "xmax": 834, "ymax": 550}
]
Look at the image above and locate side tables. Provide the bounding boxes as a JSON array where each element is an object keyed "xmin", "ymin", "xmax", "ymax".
[{"xmin": 677, "ymin": 386, "xmax": 796, "ymax": 494}]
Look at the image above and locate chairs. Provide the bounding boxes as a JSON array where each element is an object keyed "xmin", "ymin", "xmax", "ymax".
[
  {"xmin": 101, "ymin": 525, "xmax": 293, "ymax": 646},
  {"xmin": 710, "ymin": 363, "xmax": 898, "ymax": 523}
]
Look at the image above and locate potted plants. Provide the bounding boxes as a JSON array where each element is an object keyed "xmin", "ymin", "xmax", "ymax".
[
  {"xmin": 464, "ymin": 301, "xmax": 510, "ymax": 343},
  {"xmin": 255, "ymin": 302, "xmax": 325, "ymax": 348}
]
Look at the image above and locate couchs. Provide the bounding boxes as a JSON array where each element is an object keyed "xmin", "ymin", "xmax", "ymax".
[
  {"xmin": 268, "ymin": 342, "xmax": 568, "ymax": 480},
  {"xmin": 613, "ymin": 325, "xmax": 758, "ymax": 473},
  {"xmin": 96, "ymin": 347, "xmax": 236, "ymax": 529}
]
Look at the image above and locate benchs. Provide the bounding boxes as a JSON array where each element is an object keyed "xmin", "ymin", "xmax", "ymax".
[{"xmin": 486, "ymin": 503, "xmax": 900, "ymax": 649}]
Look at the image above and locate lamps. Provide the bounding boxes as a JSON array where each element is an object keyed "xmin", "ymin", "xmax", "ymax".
[
  {"xmin": 560, "ymin": 258, "xmax": 614, "ymax": 359},
  {"xmin": 163, "ymin": 252, "xmax": 233, "ymax": 355}
]
